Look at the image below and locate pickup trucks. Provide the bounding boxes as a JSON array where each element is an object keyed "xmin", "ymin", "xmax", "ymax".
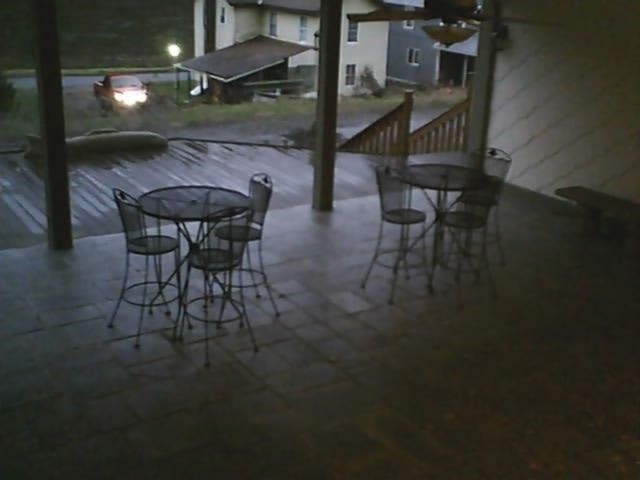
[{"xmin": 94, "ymin": 72, "xmax": 150, "ymax": 115}]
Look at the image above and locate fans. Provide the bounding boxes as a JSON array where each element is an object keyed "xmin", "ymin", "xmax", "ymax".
[{"xmin": 347, "ymin": 0, "xmax": 567, "ymax": 51}]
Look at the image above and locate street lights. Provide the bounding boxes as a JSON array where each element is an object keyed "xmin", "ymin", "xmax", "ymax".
[{"xmin": 169, "ymin": 44, "xmax": 180, "ymax": 100}]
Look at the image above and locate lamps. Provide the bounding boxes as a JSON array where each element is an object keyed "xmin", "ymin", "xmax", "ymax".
[{"xmin": 424, "ymin": 0, "xmax": 478, "ymax": 49}]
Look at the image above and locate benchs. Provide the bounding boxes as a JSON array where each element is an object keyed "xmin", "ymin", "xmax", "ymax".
[{"xmin": 554, "ymin": 185, "xmax": 639, "ymax": 253}]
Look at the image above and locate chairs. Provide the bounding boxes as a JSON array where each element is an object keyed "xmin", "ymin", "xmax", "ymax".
[
  {"xmin": 361, "ymin": 147, "xmax": 512, "ymax": 305},
  {"xmin": 108, "ymin": 174, "xmax": 280, "ymax": 367}
]
